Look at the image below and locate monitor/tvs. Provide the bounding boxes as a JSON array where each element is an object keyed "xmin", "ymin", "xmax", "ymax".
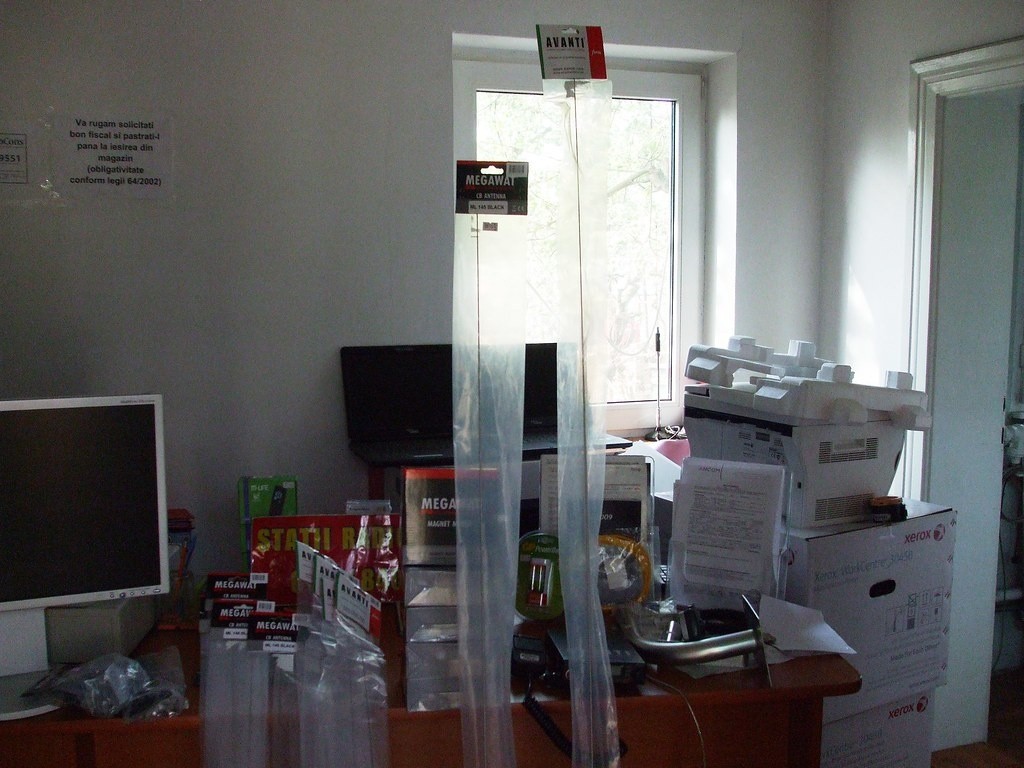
[{"xmin": 0, "ymin": 394, "xmax": 170, "ymax": 721}]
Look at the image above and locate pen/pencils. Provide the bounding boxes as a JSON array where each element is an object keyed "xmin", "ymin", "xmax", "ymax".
[
  {"xmin": 185, "ymin": 535, "xmax": 197, "ymax": 569},
  {"xmin": 174, "ymin": 541, "xmax": 188, "ymax": 596}
]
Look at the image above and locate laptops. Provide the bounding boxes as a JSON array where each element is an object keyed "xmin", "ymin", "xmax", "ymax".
[
  {"xmin": 340, "ymin": 344, "xmax": 456, "ymax": 468},
  {"xmin": 522, "ymin": 343, "xmax": 634, "ymax": 457}
]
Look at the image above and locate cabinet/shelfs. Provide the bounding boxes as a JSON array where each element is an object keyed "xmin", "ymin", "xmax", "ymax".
[{"xmin": 0, "ymin": 601, "xmax": 863, "ymax": 768}]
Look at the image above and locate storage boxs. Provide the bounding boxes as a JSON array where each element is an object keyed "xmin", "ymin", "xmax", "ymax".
[
  {"xmin": 656, "ymin": 392, "xmax": 959, "ymax": 768},
  {"xmin": 383, "ymin": 460, "xmax": 544, "ymax": 712},
  {"xmin": 46, "ymin": 593, "xmax": 155, "ymax": 664},
  {"xmin": 238, "ymin": 477, "xmax": 298, "ymax": 573}
]
